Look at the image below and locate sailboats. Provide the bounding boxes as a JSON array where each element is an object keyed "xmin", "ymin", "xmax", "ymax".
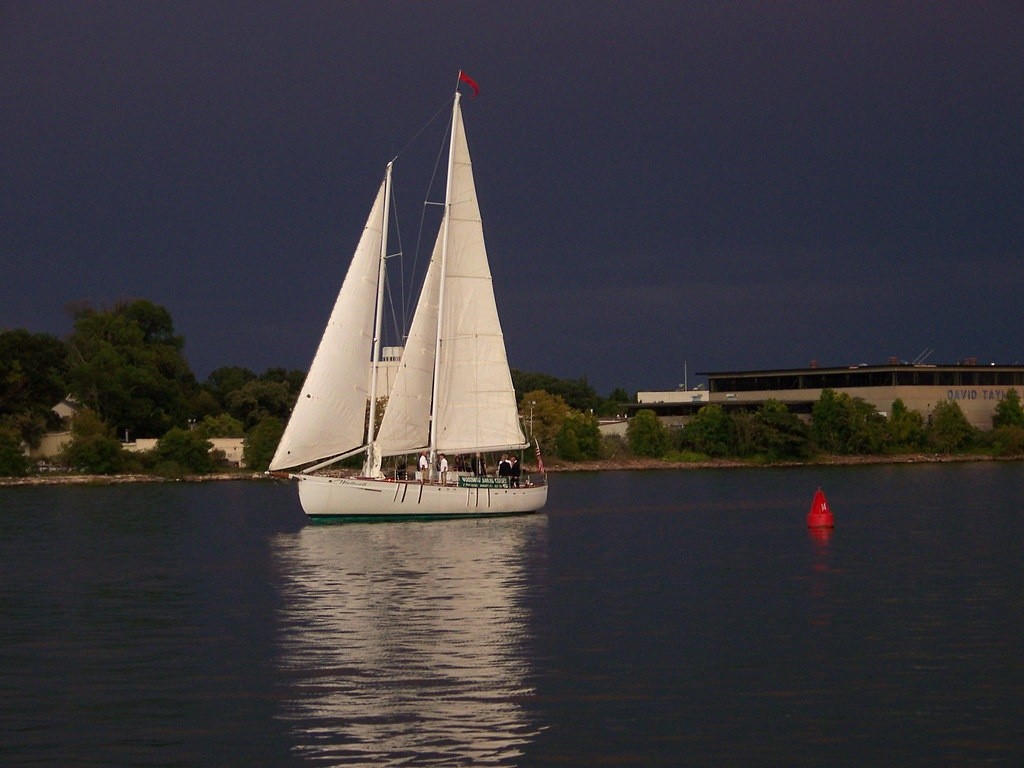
[{"xmin": 266, "ymin": 91, "xmax": 550, "ymax": 524}]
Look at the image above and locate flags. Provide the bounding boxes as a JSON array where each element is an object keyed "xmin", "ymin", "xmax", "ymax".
[{"xmin": 536, "ymin": 449, "xmax": 545, "ymax": 474}]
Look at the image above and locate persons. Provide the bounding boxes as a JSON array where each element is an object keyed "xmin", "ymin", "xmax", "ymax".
[
  {"xmin": 498, "ymin": 454, "xmax": 520, "ymax": 486},
  {"xmin": 439, "ymin": 454, "xmax": 448, "ymax": 482},
  {"xmin": 471, "ymin": 454, "xmax": 486, "ymax": 476},
  {"xmin": 420, "ymin": 451, "xmax": 428, "ymax": 479}
]
